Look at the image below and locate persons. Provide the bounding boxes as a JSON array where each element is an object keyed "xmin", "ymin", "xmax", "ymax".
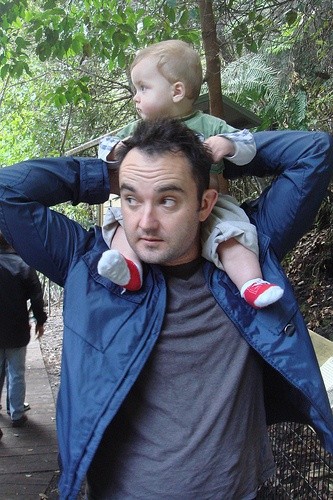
[
  {"xmin": 1, "ymin": 117, "xmax": 333, "ymax": 500},
  {"xmin": 0, "ymin": 231, "xmax": 48, "ymax": 443},
  {"xmin": 2, "ymin": 362, "xmax": 32, "ymax": 414},
  {"xmin": 96, "ymin": 40, "xmax": 284, "ymax": 310}
]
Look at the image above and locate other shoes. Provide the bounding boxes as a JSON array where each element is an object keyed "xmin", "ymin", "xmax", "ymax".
[{"xmin": 11, "ymin": 414, "xmax": 28, "ymax": 427}]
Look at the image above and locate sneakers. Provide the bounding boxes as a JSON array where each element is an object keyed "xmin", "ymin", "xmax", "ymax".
[{"xmin": 6, "ymin": 402, "xmax": 29, "ymax": 415}]
